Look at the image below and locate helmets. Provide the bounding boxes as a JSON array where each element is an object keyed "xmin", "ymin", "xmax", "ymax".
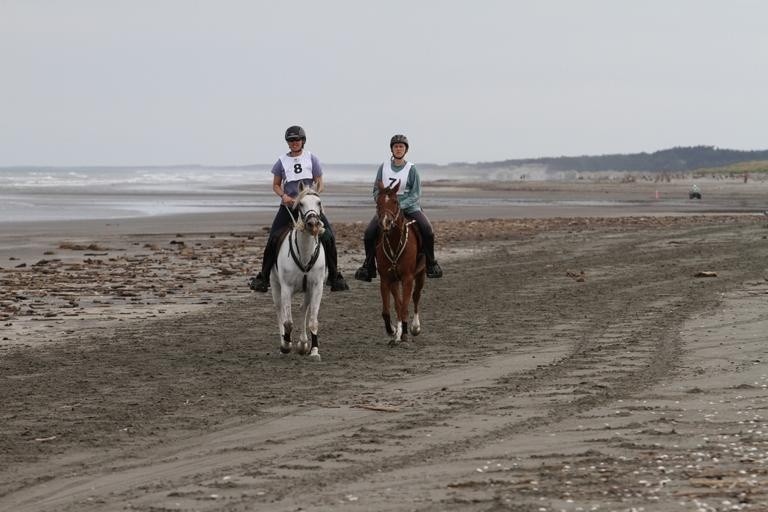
[
  {"xmin": 284, "ymin": 126, "xmax": 306, "ymax": 138},
  {"xmin": 390, "ymin": 135, "xmax": 409, "ymax": 148}
]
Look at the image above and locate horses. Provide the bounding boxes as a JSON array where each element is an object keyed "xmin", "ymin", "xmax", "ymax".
[
  {"xmin": 373, "ymin": 178, "xmax": 428, "ymax": 347},
  {"xmin": 269, "ymin": 179, "xmax": 329, "ymax": 362}
]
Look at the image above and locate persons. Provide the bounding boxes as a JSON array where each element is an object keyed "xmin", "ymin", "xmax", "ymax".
[
  {"xmin": 249, "ymin": 124, "xmax": 347, "ymax": 293},
  {"xmin": 354, "ymin": 136, "xmax": 442, "ymax": 279},
  {"xmin": 691, "ymin": 184, "xmax": 699, "ymax": 192}
]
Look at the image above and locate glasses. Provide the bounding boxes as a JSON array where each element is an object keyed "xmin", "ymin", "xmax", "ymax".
[{"xmin": 288, "ymin": 138, "xmax": 300, "ymax": 142}]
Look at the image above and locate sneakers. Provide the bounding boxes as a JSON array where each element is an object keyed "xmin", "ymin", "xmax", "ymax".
[
  {"xmin": 354, "ymin": 257, "xmax": 377, "ymax": 282},
  {"xmin": 249, "ymin": 271, "xmax": 271, "ymax": 292},
  {"xmin": 426, "ymin": 260, "xmax": 443, "ymax": 278},
  {"xmin": 326, "ymin": 271, "xmax": 349, "ymax": 292}
]
